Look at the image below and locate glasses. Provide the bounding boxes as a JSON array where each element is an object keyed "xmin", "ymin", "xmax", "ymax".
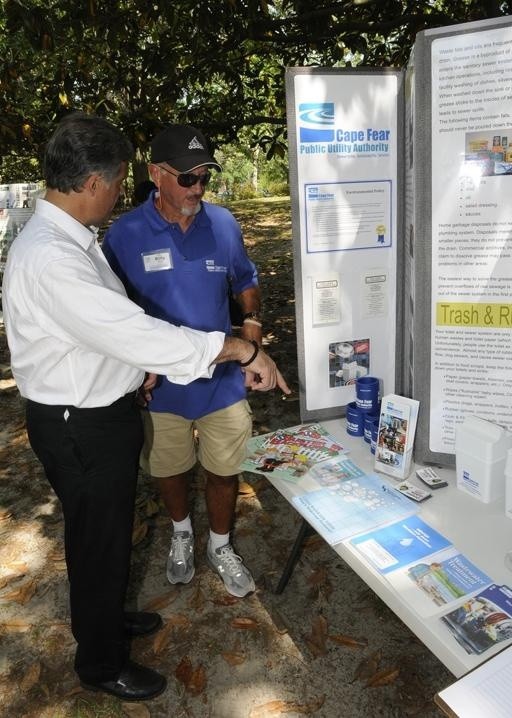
[{"xmin": 158, "ymin": 166, "xmax": 211, "ymax": 188}]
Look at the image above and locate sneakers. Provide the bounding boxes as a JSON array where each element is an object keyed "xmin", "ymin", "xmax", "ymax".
[
  {"xmin": 207, "ymin": 538, "xmax": 256, "ymax": 598},
  {"xmin": 166, "ymin": 530, "xmax": 196, "ymax": 586}
]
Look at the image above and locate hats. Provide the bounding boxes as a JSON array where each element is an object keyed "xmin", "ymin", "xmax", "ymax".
[{"xmin": 151, "ymin": 124, "xmax": 223, "ymax": 174}]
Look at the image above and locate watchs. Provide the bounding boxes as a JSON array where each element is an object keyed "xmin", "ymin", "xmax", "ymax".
[{"xmin": 242, "ymin": 311, "xmax": 265, "ymax": 325}]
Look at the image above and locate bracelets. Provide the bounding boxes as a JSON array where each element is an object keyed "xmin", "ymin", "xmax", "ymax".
[
  {"xmin": 234, "ymin": 339, "xmax": 259, "ymax": 367},
  {"xmin": 243, "ymin": 319, "xmax": 263, "ymax": 328}
]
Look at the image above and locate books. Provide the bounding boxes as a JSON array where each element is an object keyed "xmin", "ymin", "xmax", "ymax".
[
  {"xmin": 291, "ymin": 471, "xmax": 422, "ymax": 547},
  {"xmin": 373, "ymin": 393, "xmax": 421, "ymax": 480},
  {"xmin": 440, "ymin": 583, "xmax": 512, "ymax": 656}
]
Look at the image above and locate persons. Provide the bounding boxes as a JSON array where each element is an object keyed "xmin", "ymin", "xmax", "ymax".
[
  {"xmin": 2, "ymin": 116, "xmax": 293, "ymax": 701},
  {"xmin": 103, "ymin": 123, "xmax": 264, "ymax": 599}
]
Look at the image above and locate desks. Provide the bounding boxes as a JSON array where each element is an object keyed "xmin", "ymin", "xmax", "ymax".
[{"xmin": 245, "ymin": 415, "xmax": 512, "ymax": 718}]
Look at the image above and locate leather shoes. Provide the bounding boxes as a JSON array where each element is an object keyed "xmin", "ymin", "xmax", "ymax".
[
  {"xmin": 79, "ymin": 663, "xmax": 167, "ymax": 701},
  {"xmin": 124, "ymin": 611, "xmax": 161, "ymax": 637}
]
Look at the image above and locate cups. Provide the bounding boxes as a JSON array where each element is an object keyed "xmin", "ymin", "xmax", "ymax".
[{"xmin": 344, "ymin": 376, "xmax": 379, "ymax": 454}]
